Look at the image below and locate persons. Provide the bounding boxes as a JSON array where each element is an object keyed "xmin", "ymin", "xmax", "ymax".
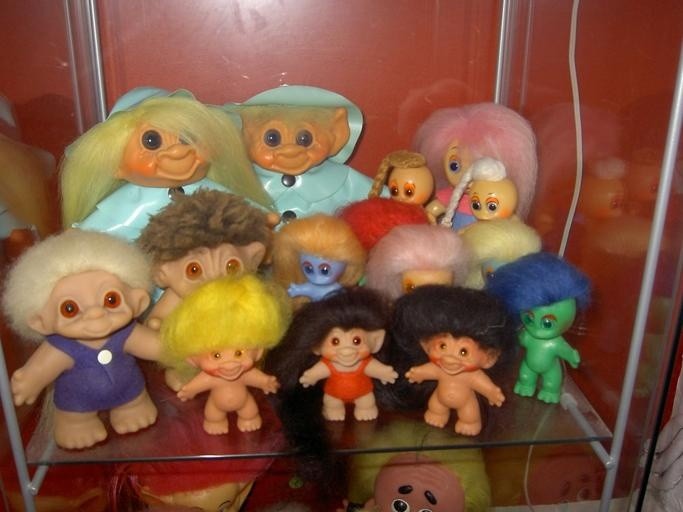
[
  {"xmin": 1, "ymin": 79, "xmax": 597, "ymax": 509},
  {"xmin": 532, "ymin": 96, "xmax": 683, "ymax": 509},
  {"xmin": 0, "ymin": 78, "xmax": 596, "ymax": 510}
]
